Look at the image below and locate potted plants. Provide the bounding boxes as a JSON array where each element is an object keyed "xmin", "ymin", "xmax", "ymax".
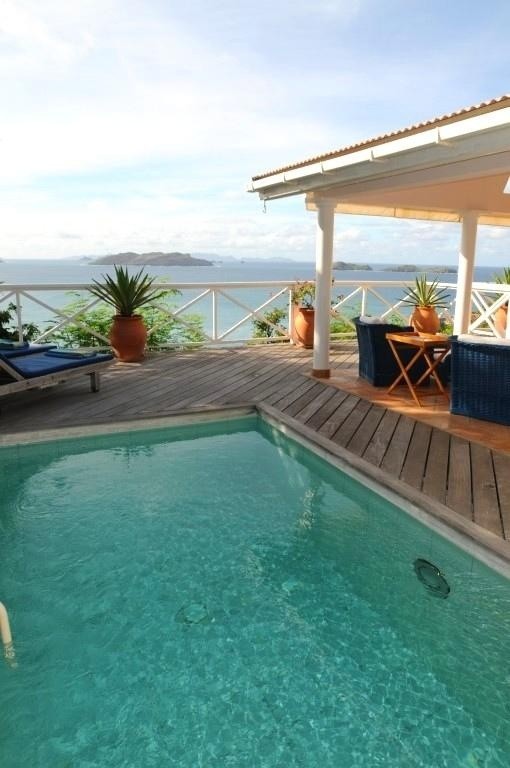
[
  {"xmin": 397, "ymin": 272, "xmax": 451, "ymax": 333},
  {"xmin": 487, "ymin": 266, "xmax": 510, "ymax": 339},
  {"xmin": 86, "ymin": 261, "xmax": 170, "ymax": 362},
  {"xmin": 287, "ymin": 276, "xmax": 346, "ymax": 349}
]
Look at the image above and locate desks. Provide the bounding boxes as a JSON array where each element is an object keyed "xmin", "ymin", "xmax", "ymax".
[{"xmin": 384, "ymin": 329, "xmax": 452, "ymax": 405}]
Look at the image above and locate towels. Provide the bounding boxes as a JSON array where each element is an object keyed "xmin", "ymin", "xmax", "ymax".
[
  {"xmin": 0, "ymin": 339, "xmax": 30, "ymax": 351},
  {"xmin": 44, "ymin": 347, "xmax": 97, "ymax": 360}
]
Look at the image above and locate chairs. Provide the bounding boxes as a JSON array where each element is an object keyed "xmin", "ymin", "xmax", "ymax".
[
  {"xmin": 0, "ymin": 338, "xmax": 57, "ymax": 358},
  {"xmin": 352, "ymin": 314, "xmax": 431, "ymax": 387},
  {"xmin": 446, "ymin": 333, "xmax": 510, "ymax": 427},
  {"xmin": 0, "ymin": 350, "xmax": 119, "ymax": 396}
]
